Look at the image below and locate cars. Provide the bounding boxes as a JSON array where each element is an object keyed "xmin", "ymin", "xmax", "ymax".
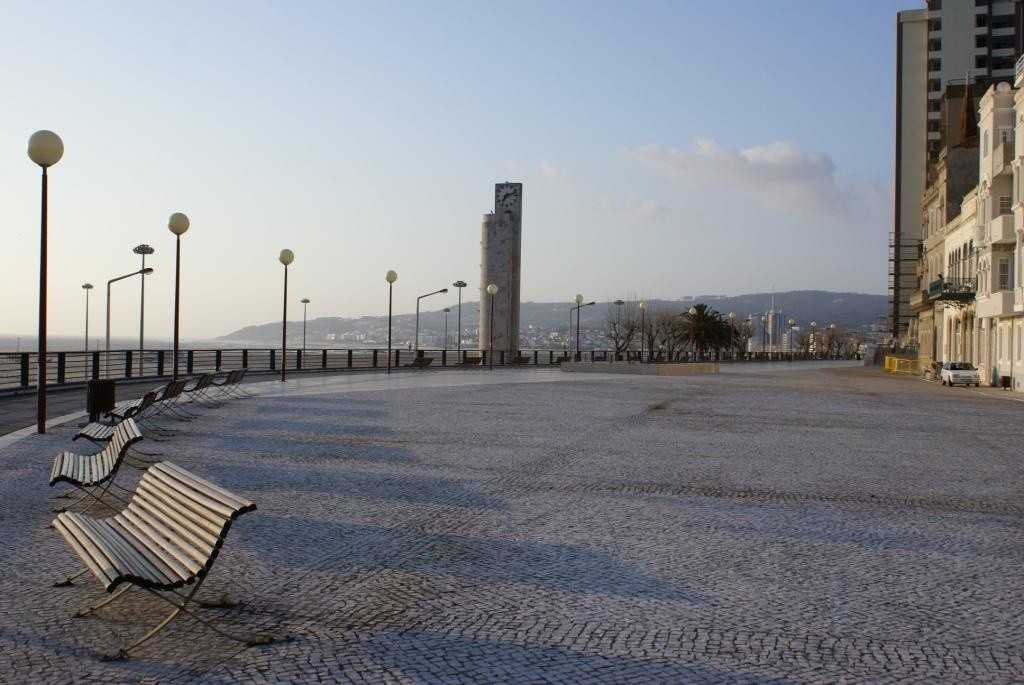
[{"xmin": 941, "ymin": 360, "xmax": 980, "ymax": 387}]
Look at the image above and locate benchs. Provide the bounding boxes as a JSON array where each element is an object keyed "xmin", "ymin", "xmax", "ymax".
[
  {"xmin": 594, "ymin": 356, "xmax": 607, "ymax": 361},
  {"xmin": 104, "ymin": 385, "xmax": 175, "ymax": 443},
  {"xmin": 52, "ymin": 461, "xmax": 274, "ymax": 661},
  {"xmin": 182, "ymin": 371, "xmax": 226, "ymax": 409},
  {"xmin": 550, "ymin": 357, "xmax": 571, "ymax": 364},
  {"xmin": 404, "ymin": 358, "xmax": 433, "ymax": 367},
  {"xmin": 506, "ymin": 357, "xmax": 530, "ymax": 364},
  {"xmin": 456, "ymin": 357, "xmax": 482, "ymax": 365},
  {"xmin": 154, "ymin": 378, "xmax": 195, "ymax": 422},
  {"xmin": 49, "ymin": 417, "xmax": 137, "ymax": 529},
  {"xmin": 73, "ymin": 421, "xmax": 160, "ymax": 471},
  {"xmin": 207, "ymin": 368, "xmax": 257, "ymax": 400}
]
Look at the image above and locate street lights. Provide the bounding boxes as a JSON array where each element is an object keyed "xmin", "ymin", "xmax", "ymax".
[
  {"xmin": 688, "ymin": 306, "xmax": 698, "ymax": 362},
  {"xmin": 300, "ymin": 298, "xmax": 310, "ymax": 368},
  {"xmin": 26, "ymin": 129, "xmax": 66, "ymax": 435},
  {"xmin": 761, "ymin": 315, "xmax": 769, "ymax": 352},
  {"xmin": 105, "ymin": 268, "xmax": 154, "ymax": 380},
  {"xmin": 168, "ymin": 212, "xmax": 190, "ymax": 381},
  {"xmin": 569, "ymin": 301, "xmax": 596, "ymax": 351},
  {"xmin": 810, "ymin": 321, "xmax": 817, "ymax": 352},
  {"xmin": 415, "ymin": 288, "xmax": 449, "ymax": 350},
  {"xmin": 830, "ymin": 323, "xmax": 837, "ymax": 353},
  {"xmin": 788, "ymin": 319, "xmax": 796, "ymax": 353},
  {"xmin": 385, "ymin": 270, "xmax": 398, "ymax": 374},
  {"xmin": 573, "ymin": 293, "xmax": 584, "ymax": 354},
  {"xmin": 453, "ymin": 280, "xmax": 468, "ymax": 364},
  {"xmin": 82, "ymin": 283, "xmax": 94, "ymax": 380},
  {"xmin": 728, "ymin": 311, "xmax": 737, "ymax": 357},
  {"xmin": 443, "ymin": 307, "xmax": 450, "ymax": 350},
  {"xmin": 278, "ymin": 248, "xmax": 295, "ymax": 381},
  {"xmin": 486, "ymin": 284, "xmax": 499, "ymax": 370},
  {"xmin": 132, "ymin": 244, "xmax": 154, "ymax": 376},
  {"xmin": 639, "ymin": 302, "xmax": 649, "ymax": 361}
]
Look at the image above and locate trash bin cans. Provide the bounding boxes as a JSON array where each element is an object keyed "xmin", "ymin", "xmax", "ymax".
[
  {"xmin": 86, "ymin": 378, "xmax": 116, "ymax": 412},
  {"xmin": 1000, "ymin": 376, "xmax": 1010, "ymax": 388}
]
